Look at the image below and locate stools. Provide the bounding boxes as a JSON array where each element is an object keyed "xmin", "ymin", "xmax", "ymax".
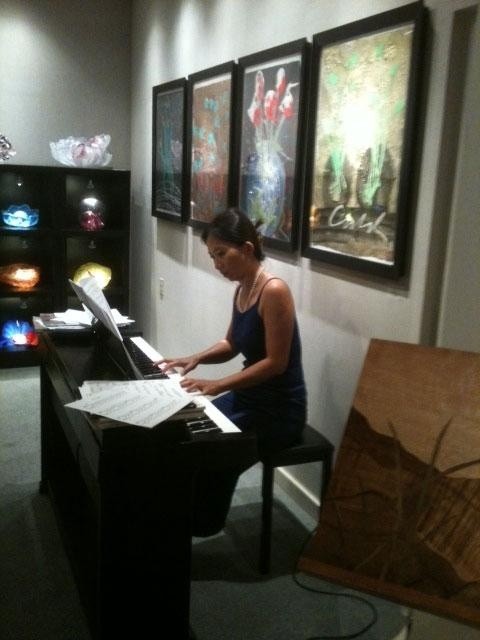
[{"xmin": 257, "ymin": 423, "xmax": 337, "ymax": 576}]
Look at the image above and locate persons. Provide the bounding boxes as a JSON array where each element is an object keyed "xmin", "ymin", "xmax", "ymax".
[{"xmin": 150, "ymin": 207, "xmax": 308, "ymax": 537}]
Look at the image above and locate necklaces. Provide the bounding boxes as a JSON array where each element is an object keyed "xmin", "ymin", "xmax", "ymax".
[{"xmin": 237, "ymin": 266, "xmax": 265, "ymax": 315}]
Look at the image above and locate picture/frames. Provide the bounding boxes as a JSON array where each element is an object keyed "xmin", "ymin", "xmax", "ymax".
[
  {"xmin": 300, "ymin": 0, "xmax": 433, "ymax": 291},
  {"xmin": 150, "ymin": 77, "xmax": 188, "ymax": 226},
  {"xmin": 243, "ymin": 37, "xmax": 311, "ymax": 255},
  {"xmin": 186, "ymin": 61, "xmax": 238, "ymax": 231}
]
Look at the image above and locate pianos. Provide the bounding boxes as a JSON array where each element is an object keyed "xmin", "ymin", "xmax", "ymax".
[{"xmin": 38, "ymin": 329, "xmax": 255, "ymax": 637}]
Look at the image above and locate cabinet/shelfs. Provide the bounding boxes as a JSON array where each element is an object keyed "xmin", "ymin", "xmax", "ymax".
[{"xmin": 0, "ymin": 165, "xmax": 135, "ymax": 372}]
